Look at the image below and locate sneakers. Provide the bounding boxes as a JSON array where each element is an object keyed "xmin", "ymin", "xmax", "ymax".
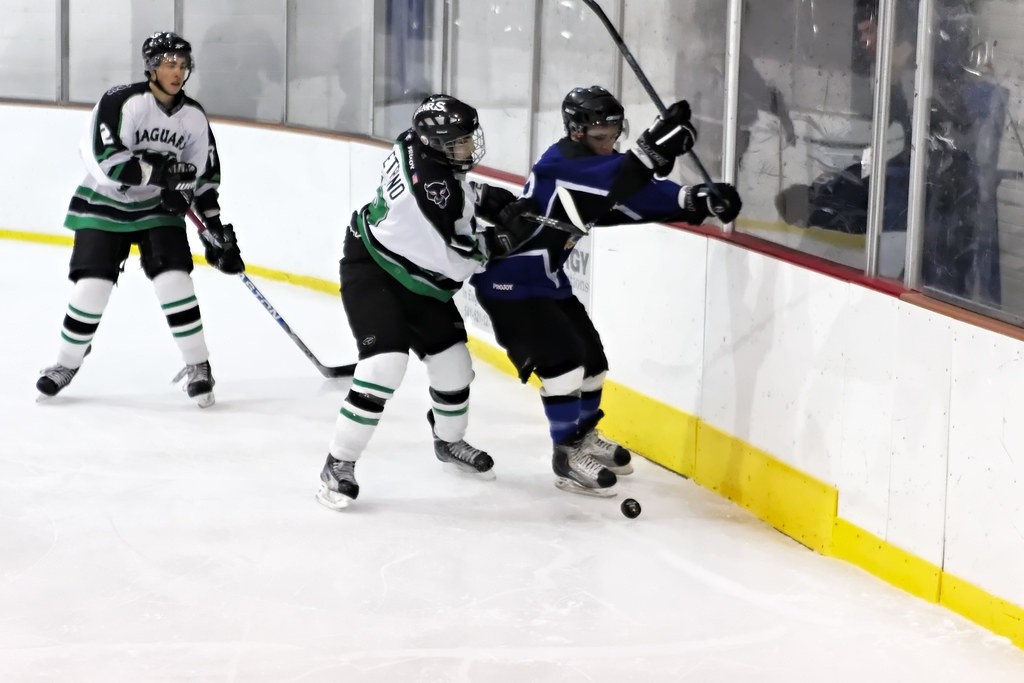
[
  {"xmin": 172, "ymin": 361, "xmax": 215, "ymax": 397},
  {"xmin": 435, "ymin": 441, "xmax": 494, "ymax": 472},
  {"xmin": 37, "ymin": 364, "xmax": 80, "ymax": 395},
  {"xmin": 552, "ymin": 409, "xmax": 631, "ymax": 487},
  {"xmin": 320, "ymin": 454, "xmax": 359, "ymax": 499}
]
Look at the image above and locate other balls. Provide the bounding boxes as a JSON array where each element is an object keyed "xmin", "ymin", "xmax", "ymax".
[{"xmin": 620, "ymin": 496, "xmax": 644, "ymax": 518}]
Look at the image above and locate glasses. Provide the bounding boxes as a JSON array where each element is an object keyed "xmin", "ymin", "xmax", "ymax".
[{"xmin": 584, "ymin": 133, "xmax": 619, "ymax": 141}]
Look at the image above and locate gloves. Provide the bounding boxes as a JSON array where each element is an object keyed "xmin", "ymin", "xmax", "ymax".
[
  {"xmin": 204, "ymin": 223, "xmax": 245, "ymax": 275},
  {"xmin": 481, "ymin": 183, "xmax": 516, "ymax": 224},
  {"xmin": 636, "ymin": 100, "xmax": 698, "ymax": 178},
  {"xmin": 684, "ymin": 183, "xmax": 741, "ymax": 226},
  {"xmin": 486, "ymin": 199, "xmax": 543, "ymax": 258},
  {"xmin": 161, "ymin": 159, "xmax": 197, "ymax": 218}
]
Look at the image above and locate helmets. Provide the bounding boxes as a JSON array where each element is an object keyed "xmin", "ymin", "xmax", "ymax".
[
  {"xmin": 143, "ymin": 32, "xmax": 193, "ymax": 72},
  {"xmin": 413, "ymin": 95, "xmax": 486, "ymax": 179},
  {"xmin": 563, "ymin": 86, "xmax": 624, "ymax": 128}
]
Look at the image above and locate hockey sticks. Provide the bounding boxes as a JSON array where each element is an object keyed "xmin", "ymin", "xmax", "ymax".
[
  {"xmin": 516, "ymin": 186, "xmax": 589, "ymax": 237},
  {"xmin": 582, "ymin": 0, "xmax": 730, "ymax": 211},
  {"xmin": 183, "ymin": 204, "xmax": 360, "ymax": 378}
]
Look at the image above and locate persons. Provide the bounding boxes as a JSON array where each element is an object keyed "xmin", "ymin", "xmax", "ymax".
[
  {"xmin": 676, "ymin": 1, "xmax": 797, "ymax": 225},
  {"xmin": 468, "ymin": 85, "xmax": 743, "ymax": 498},
  {"xmin": 314, "ymin": 93, "xmax": 541, "ymax": 512},
  {"xmin": 35, "ymin": 32, "xmax": 244, "ymax": 410},
  {"xmin": 772, "ymin": 7, "xmax": 978, "ymax": 298}
]
